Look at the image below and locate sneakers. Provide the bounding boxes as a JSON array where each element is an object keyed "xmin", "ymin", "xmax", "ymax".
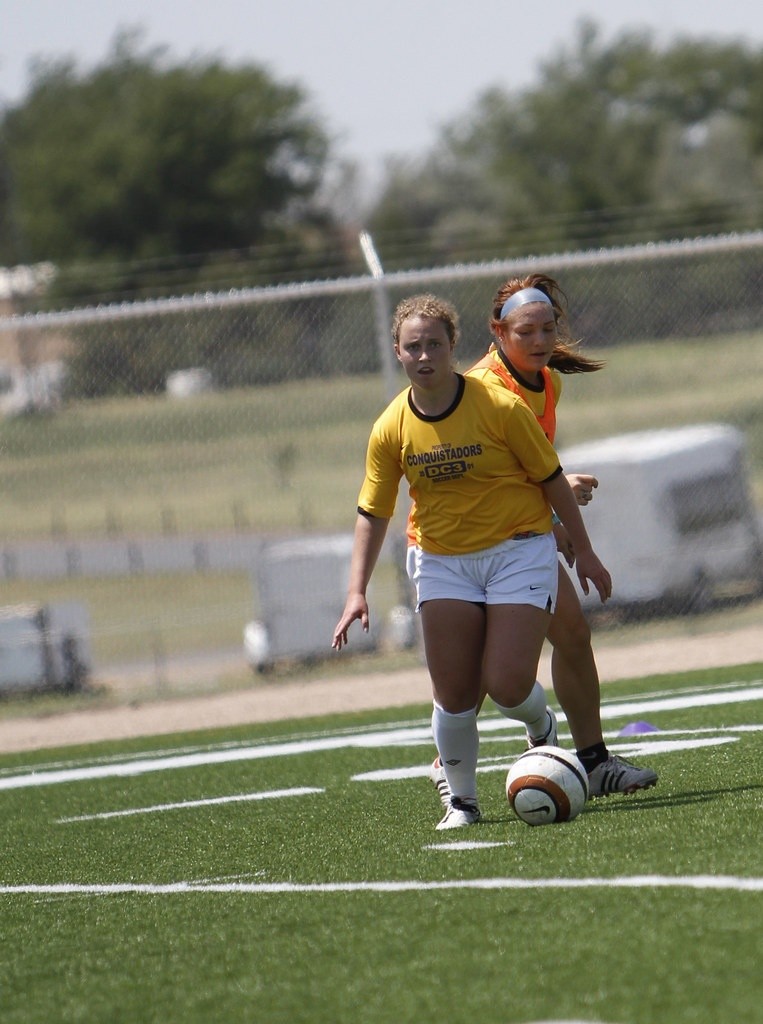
[
  {"xmin": 435, "ymin": 797, "xmax": 481, "ymax": 829},
  {"xmin": 586, "ymin": 751, "xmax": 658, "ymax": 799},
  {"xmin": 524, "ymin": 705, "xmax": 558, "ymax": 751},
  {"xmin": 428, "ymin": 755, "xmax": 452, "ymax": 806}
]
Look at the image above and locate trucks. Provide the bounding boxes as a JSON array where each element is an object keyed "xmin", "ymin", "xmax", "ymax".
[
  {"xmin": 257, "ymin": 532, "xmax": 372, "ymax": 674},
  {"xmin": 549, "ymin": 424, "xmax": 763, "ymax": 617}
]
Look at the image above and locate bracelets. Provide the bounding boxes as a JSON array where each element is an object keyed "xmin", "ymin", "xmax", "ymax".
[{"xmin": 552, "ymin": 513, "xmax": 561, "ymax": 526}]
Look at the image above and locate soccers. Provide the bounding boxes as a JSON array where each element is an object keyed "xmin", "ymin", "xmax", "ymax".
[{"xmin": 505, "ymin": 744, "xmax": 590, "ymax": 827}]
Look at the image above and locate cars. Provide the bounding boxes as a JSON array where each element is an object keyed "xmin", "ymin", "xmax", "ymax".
[{"xmin": 0, "ymin": 604, "xmax": 91, "ymax": 692}]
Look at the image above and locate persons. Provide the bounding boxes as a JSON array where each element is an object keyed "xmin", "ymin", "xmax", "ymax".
[
  {"xmin": 402, "ymin": 273, "xmax": 661, "ymax": 799},
  {"xmin": 329, "ymin": 292, "xmax": 615, "ymax": 832}
]
